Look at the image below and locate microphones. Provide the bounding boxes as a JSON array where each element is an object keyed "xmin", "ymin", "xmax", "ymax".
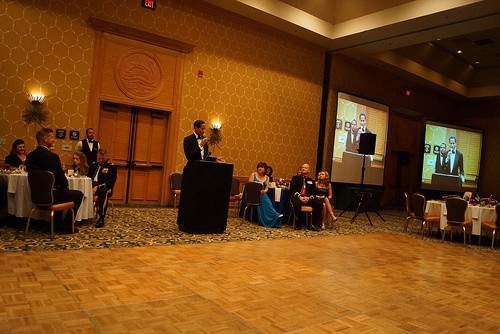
[{"xmin": 203, "ymin": 133, "xmax": 208, "ymax": 146}]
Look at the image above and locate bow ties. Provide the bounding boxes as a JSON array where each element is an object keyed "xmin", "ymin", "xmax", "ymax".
[
  {"xmin": 198, "ymin": 135, "xmax": 205, "ymax": 139},
  {"xmin": 448, "ymin": 150, "xmax": 455, "ymax": 154},
  {"xmin": 88, "ymin": 140, "xmax": 93, "ymax": 143}
]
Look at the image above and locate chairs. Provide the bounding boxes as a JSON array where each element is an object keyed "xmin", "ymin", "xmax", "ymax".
[
  {"xmin": 410, "ymin": 194, "xmax": 440, "ymax": 240},
  {"xmin": 168, "ymin": 173, "xmax": 183, "ymax": 209},
  {"xmin": 288, "ymin": 199, "xmax": 313, "ymax": 231},
  {"xmin": 229, "ymin": 178, "xmax": 242, "ymax": 217},
  {"xmin": 25, "ymin": 170, "xmax": 75, "ymax": 241},
  {"xmin": 442, "ymin": 197, "xmax": 473, "ymax": 245},
  {"xmin": 403, "ymin": 193, "xmax": 417, "ymax": 233},
  {"xmin": 243, "ymin": 182, "xmax": 262, "ymax": 224},
  {"xmin": 479, "ymin": 203, "xmax": 500, "ymax": 249},
  {"xmin": 93, "ymin": 174, "xmax": 119, "ymax": 220}
]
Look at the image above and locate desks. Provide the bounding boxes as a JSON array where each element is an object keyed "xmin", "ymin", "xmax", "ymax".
[
  {"xmin": 239, "ymin": 188, "xmax": 294, "ymax": 225},
  {"xmin": 0, "ymin": 171, "xmax": 94, "ymax": 221},
  {"xmin": 426, "ymin": 200, "xmax": 496, "ymax": 235}
]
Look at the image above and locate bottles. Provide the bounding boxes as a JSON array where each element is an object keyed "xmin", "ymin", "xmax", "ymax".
[
  {"xmin": 465, "ymin": 195, "xmax": 493, "ymax": 207},
  {"xmin": 441, "ymin": 194, "xmax": 448, "ymax": 203}
]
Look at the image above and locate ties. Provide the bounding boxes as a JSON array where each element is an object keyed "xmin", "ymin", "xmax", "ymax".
[{"xmin": 92, "ymin": 165, "xmax": 99, "ymax": 180}]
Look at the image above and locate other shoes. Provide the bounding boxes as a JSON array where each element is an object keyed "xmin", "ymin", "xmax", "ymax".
[
  {"xmin": 321, "ymin": 223, "xmax": 326, "ymax": 230},
  {"xmin": 54, "ymin": 223, "xmax": 79, "ymax": 234},
  {"xmin": 294, "ymin": 226, "xmax": 302, "ymax": 230},
  {"xmin": 95, "ymin": 217, "xmax": 104, "ymax": 228},
  {"xmin": 308, "ymin": 225, "xmax": 317, "ymax": 231},
  {"xmin": 333, "ymin": 217, "xmax": 338, "ymax": 222}
]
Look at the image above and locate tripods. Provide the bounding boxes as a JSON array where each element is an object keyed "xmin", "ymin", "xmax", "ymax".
[{"xmin": 338, "ymin": 154, "xmax": 386, "ymax": 226}]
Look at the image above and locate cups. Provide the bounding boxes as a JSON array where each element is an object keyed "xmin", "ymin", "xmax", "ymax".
[
  {"xmin": 271, "ymin": 182, "xmax": 276, "ymax": 187},
  {"xmin": 2, "ymin": 164, "xmax": 26, "ymax": 173},
  {"xmin": 68, "ymin": 169, "xmax": 74, "ymax": 177}
]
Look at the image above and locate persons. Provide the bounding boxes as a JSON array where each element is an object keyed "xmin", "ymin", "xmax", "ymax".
[
  {"xmin": 183, "ymin": 120, "xmax": 226, "ymax": 164},
  {"xmin": 444, "ymin": 136, "xmax": 465, "ymax": 183},
  {"xmin": 25, "ymin": 128, "xmax": 84, "ymax": 235},
  {"xmin": 75, "ymin": 128, "xmax": 101, "ymax": 166},
  {"xmin": 315, "ymin": 170, "xmax": 338, "ymax": 230},
  {"xmin": 289, "ymin": 163, "xmax": 323, "ymax": 231},
  {"xmin": 240, "ymin": 162, "xmax": 283, "ymax": 228},
  {"xmin": 435, "ymin": 143, "xmax": 447, "ymax": 173},
  {"xmin": 71, "ymin": 151, "xmax": 89, "ymax": 175},
  {"xmin": 264, "ymin": 166, "xmax": 274, "ymax": 182},
  {"xmin": 345, "ymin": 119, "xmax": 358, "ymax": 152},
  {"xmin": 4, "ymin": 139, "xmax": 27, "ymax": 170},
  {"xmin": 354, "ymin": 113, "xmax": 373, "ymax": 162},
  {"xmin": 75, "ymin": 148, "xmax": 117, "ymax": 228}
]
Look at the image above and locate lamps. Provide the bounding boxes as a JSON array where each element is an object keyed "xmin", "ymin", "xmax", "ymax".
[
  {"xmin": 29, "ymin": 93, "xmax": 45, "ymax": 105},
  {"xmin": 212, "ymin": 123, "xmax": 221, "ymax": 132}
]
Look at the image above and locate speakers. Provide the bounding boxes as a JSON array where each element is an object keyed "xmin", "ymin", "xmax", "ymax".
[{"xmin": 358, "ymin": 133, "xmax": 377, "ymax": 155}]
